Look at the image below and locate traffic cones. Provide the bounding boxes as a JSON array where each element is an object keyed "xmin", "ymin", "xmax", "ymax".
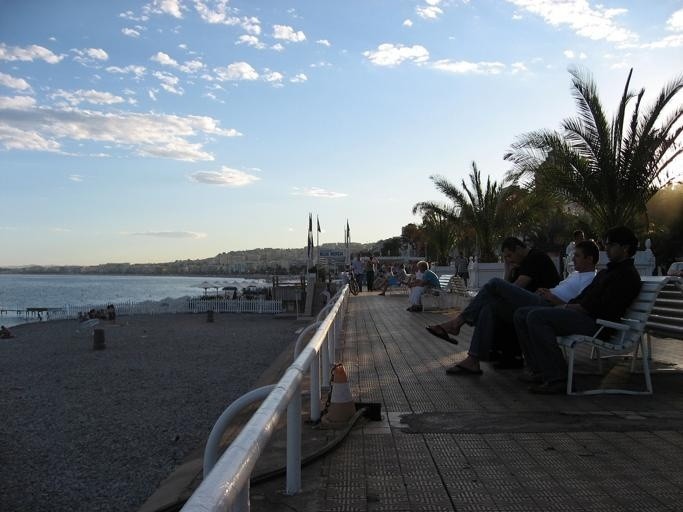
[{"xmin": 320, "ymin": 367, "xmax": 356, "ymax": 429}]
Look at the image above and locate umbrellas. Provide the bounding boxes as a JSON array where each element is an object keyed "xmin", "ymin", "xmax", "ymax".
[{"xmin": 189, "ymin": 280, "xmax": 264, "ymax": 296}]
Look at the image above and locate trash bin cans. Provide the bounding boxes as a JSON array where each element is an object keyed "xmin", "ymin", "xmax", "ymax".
[
  {"xmin": 94, "ymin": 328, "xmax": 104, "ymax": 350},
  {"xmin": 207, "ymin": 310, "xmax": 214, "ymax": 322}
]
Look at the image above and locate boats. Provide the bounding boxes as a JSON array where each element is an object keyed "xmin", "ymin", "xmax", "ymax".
[{"xmin": 198, "ymin": 280, "xmax": 272, "ymax": 301}]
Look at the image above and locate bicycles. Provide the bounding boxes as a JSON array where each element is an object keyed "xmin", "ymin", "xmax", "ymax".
[{"xmin": 346, "ymin": 268, "xmax": 360, "ymax": 295}]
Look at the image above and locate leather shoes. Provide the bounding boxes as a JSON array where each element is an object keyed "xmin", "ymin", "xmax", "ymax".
[
  {"xmin": 517, "ymin": 366, "xmax": 579, "ymax": 395},
  {"xmin": 406, "ymin": 303, "xmax": 423, "ymax": 313}
]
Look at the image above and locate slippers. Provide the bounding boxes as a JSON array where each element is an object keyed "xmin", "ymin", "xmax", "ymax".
[
  {"xmin": 445, "ymin": 356, "xmax": 484, "ymax": 377},
  {"xmin": 424, "ymin": 325, "xmax": 458, "ymax": 345}
]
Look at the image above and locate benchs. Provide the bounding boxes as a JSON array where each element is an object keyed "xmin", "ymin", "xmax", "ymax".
[
  {"xmin": 555, "ymin": 276, "xmax": 683, "ymax": 396},
  {"xmin": 374, "ymin": 267, "xmax": 478, "ymax": 314}
]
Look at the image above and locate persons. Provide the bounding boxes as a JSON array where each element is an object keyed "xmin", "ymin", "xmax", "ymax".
[
  {"xmin": 404, "ymin": 259, "xmax": 440, "ymax": 312},
  {"xmin": 77, "ymin": 303, "xmax": 117, "ymax": 324},
  {"xmin": 0, "ymin": 325, "xmax": 16, "ymax": 339},
  {"xmin": 425, "ymin": 226, "xmax": 642, "ymax": 395},
  {"xmin": 353, "ymin": 254, "xmax": 404, "ymax": 295}
]
